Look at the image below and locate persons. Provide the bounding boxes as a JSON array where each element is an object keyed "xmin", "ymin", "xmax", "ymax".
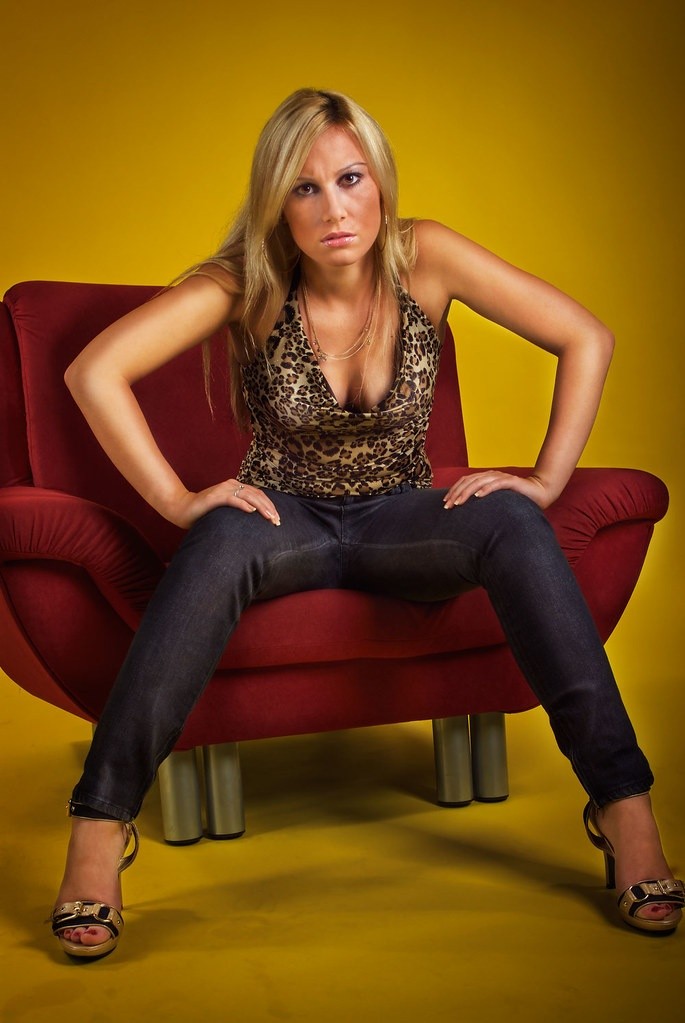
[{"xmin": 46, "ymin": 86, "xmax": 682, "ymax": 963}]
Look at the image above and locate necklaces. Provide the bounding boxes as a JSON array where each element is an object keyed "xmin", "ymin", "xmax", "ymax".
[{"xmin": 302, "ymin": 280, "xmax": 380, "ymax": 360}]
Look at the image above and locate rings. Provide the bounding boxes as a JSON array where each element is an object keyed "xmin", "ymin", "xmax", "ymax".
[
  {"xmin": 237, "ymin": 485, "xmax": 244, "ymax": 491},
  {"xmin": 233, "ymin": 489, "xmax": 240, "ymax": 498}
]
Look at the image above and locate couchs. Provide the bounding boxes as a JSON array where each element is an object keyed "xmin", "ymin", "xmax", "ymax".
[{"xmin": 0, "ymin": 277, "xmax": 672, "ymax": 851}]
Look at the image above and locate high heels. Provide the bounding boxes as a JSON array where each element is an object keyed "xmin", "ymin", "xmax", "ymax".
[
  {"xmin": 44, "ymin": 799, "xmax": 139, "ymax": 959},
  {"xmin": 583, "ymin": 800, "xmax": 685, "ymax": 934}
]
